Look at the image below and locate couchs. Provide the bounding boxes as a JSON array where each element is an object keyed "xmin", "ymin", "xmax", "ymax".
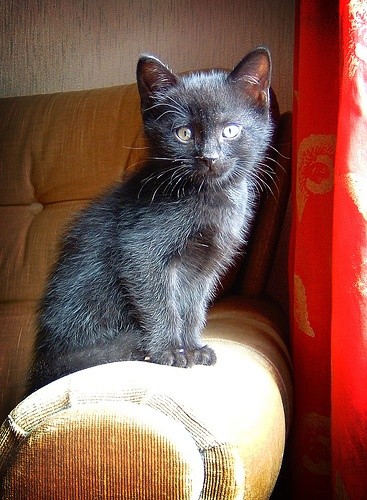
[{"xmin": 0, "ymin": 65, "xmax": 294, "ymax": 500}]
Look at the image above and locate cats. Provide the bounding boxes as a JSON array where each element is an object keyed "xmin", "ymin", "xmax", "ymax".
[{"xmin": 24, "ymin": 46, "xmax": 289, "ymax": 399}]
